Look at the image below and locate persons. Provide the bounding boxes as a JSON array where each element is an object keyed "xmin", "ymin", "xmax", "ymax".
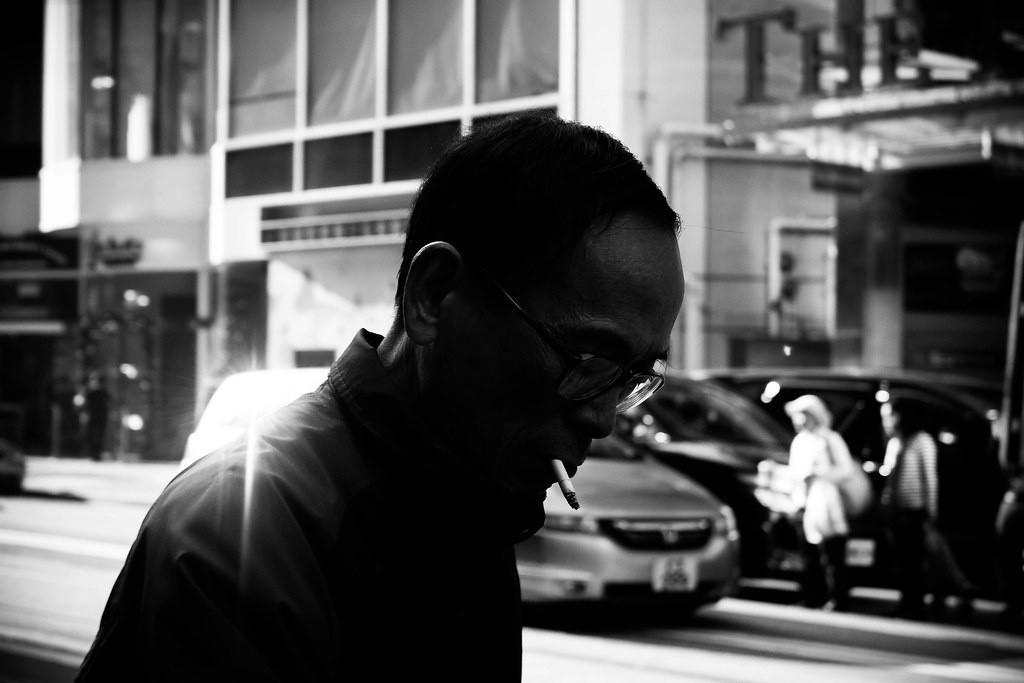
[
  {"xmin": 46, "ymin": 365, "xmax": 109, "ymax": 460},
  {"xmin": 75, "ymin": 112, "xmax": 684, "ymax": 683},
  {"xmin": 876, "ymin": 394, "xmax": 976, "ymax": 615},
  {"xmin": 781, "ymin": 395, "xmax": 873, "ymax": 608}
]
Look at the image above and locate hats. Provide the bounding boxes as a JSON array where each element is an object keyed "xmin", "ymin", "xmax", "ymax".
[{"xmin": 784, "ymin": 395, "xmax": 832, "ymax": 429}]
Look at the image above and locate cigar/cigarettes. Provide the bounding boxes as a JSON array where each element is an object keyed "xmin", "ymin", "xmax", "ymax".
[{"xmin": 552, "ymin": 458, "xmax": 580, "ymax": 511}]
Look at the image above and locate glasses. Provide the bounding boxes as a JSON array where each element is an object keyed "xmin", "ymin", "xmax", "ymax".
[{"xmin": 463, "ymin": 257, "xmax": 667, "ymax": 417}]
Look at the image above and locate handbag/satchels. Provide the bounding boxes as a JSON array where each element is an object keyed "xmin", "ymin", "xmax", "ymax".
[{"xmin": 825, "ymin": 437, "xmax": 871, "ymax": 516}]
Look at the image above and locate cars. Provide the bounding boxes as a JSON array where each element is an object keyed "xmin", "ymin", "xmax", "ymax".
[
  {"xmin": 615, "ymin": 372, "xmax": 1024, "ymax": 608},
  {"xmin": 177, "ymin": 370, "xmax": 742, "ymax": 620}
]
